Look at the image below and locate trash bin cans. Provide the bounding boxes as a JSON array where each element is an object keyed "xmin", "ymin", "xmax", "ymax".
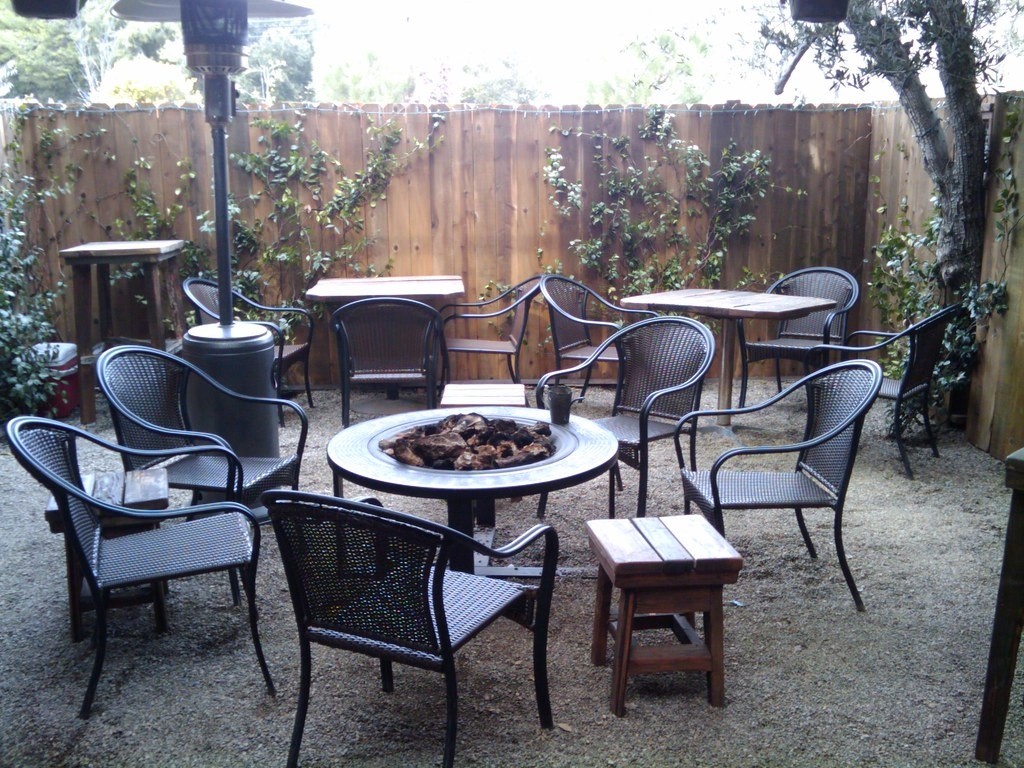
[{"xmin": 22, "ymin": 342, "xmax": 78, "ymax": 418}]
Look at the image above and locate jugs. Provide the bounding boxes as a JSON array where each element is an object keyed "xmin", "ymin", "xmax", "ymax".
[{"xmin": 538, "ymin": 384, "xmax": 586, "ymax": 425}]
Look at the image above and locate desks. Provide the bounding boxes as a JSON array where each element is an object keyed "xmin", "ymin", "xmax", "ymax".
[
  {"xmin": 618, "ymin": 288, "xmax": 838, "ymax": 438},
  {"xmin": 327, "ymin": 404, "xmax": 622, "ymax": 579},
  {"xmin": 304, "ymin": 277, "xmax": 466, "ymax": 415}
]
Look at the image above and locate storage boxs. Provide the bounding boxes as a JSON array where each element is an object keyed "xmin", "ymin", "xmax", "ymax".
[{"xmin": 18, "ymin": 341, "xmax": 82, "ymax": 419}]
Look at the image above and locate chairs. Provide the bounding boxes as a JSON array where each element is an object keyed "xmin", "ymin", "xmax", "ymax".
[
  {"xmin": 804, "ymin": 303, "xmax": 961, "ymax": 482},
  {"xmin": 96, "ymin": 346, "xmax": 308, "ymax": 522},
  {"xmin": 259, "ymin": 486, "xmax": 562, "ymax": 768},
  {"xmin": 675, "ymin": 360, "xmax": 885, "ymax": 614},
  {"xmin": 180, "ymin": 274, "xmax": 315, "ymax": 430},
  {"xmin": 535, "ymin": 312, "xmax": 715, "ymax": 521},
  {"xmin": 330, "ymin": 297, "xmax": 447, "ymax": 429},
  {"xmin": 259, "ymin": 488, "xmax": 565, "ymax": 767},
  {"xmin": 3, "ymin": 414, "xmax": 277, "ymax": 721},
  {"xmin": 735, "ymin": 267, "xmax": 860, "ymax": 410},
  {"xmin": 538, "ymin": 272, "xmax": 661, "ymax": 403},
  {"xmin": 432, "ymin": 274, "xmax": 545, "ymax": 410}
]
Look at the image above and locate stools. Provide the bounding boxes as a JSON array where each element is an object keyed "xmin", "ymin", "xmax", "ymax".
[
  {"xmin": 42, "ymin": 467, "xmax": 169, "ymax": 644},
  {"xmin": 55, "ymin": 239, "xmax": 187, "ymax": 425},
  {"xmin": 438, "ymin": 383, "xmax": 528, "ymax": 502},
  {"xmin": 973, "ymin": 450, "xmax": 1024, "ymax": 762},
  {"xmin": 585, "ymin": 512, "xmax": 742, "ymax": 718}
]
